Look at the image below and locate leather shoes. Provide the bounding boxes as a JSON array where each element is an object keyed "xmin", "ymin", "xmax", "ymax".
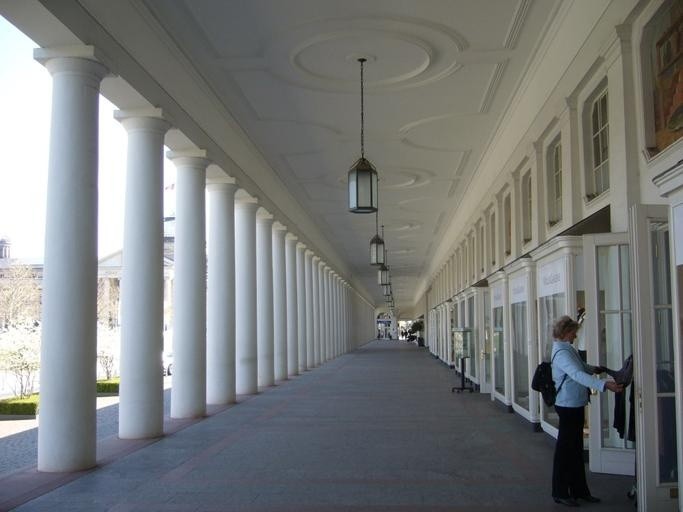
[
  {"xmin": 553, "ymin": 494, "xmax": 579, "ymax": 507},
  {"xmin": 571, "ymin": 494, "xmax": 601, "ymax": 503}
]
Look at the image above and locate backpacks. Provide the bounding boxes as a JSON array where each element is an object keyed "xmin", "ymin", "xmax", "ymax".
[{"xmin": 531, "ymin": 349, "xmax": 574, "ymax": 408}]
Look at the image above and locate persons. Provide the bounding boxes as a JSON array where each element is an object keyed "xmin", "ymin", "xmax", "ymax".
[
  {"xmin": 550, "ymin": 315, "xmax": 625, "ymax": 507},
  {"xmin": 401, "ymin": 330, "xmax": 408, "ymax": 339}
]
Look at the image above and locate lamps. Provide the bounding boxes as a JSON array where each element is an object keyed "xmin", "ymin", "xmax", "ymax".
[
  {"xmin": 382, "ymin": 285, "xmax": 391, "ymax": 296},
  {"xmin": 369, "ymin": 210, "xmax": 384, "ymax": 265},
  {"xmin": 376, "ymin": 224, "xmax": 389, "ymax": 286},
  {"xmin": 346, "ymin": 57, "xmax": 378, "ymax": 214}
]
